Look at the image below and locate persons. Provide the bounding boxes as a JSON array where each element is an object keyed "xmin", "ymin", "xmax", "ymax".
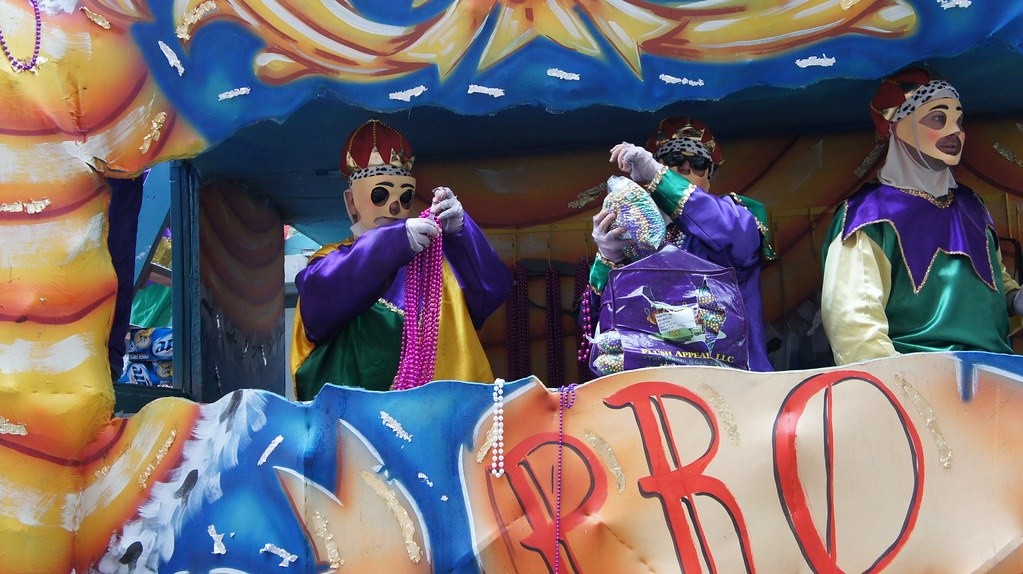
[
  {"xmin": 579, "ymin": 113, "xmax": 779, "ymax": 386},
  {"xmin": 820, "ymin": 65, "xmax": 1023, "ymax": 366},
  {"xmin": 290, "ymin": 119, "xmax": 513, "ymax": 403}
]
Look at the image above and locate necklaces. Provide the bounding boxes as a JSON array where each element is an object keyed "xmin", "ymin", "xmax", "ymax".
[
  {"xmin": 492, "ymin": 378, "xmax": 505, "ymax": 477},
  {"xmin": 392, "ymin": 208, "xmax": 443, "ymax": 390},
  {"xmin": 505, "ymin": 175, "xmax": 750, "ymax": 389},
  {"xmin": 555, "ymin": 383, "xmax": 577, "ymax": 574}
]
